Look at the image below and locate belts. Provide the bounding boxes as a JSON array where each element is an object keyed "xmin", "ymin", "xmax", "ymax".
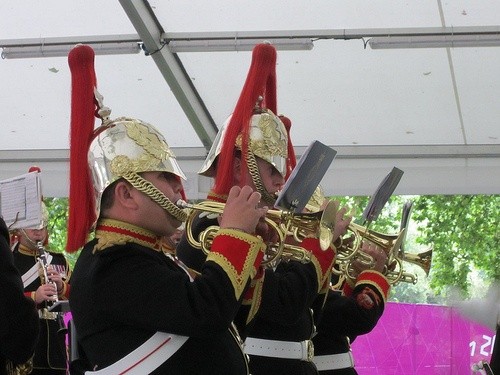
[
  {"xmin": 38, "ymin": 308, "xmax": 58, "ymax": 319},
  {"xmin": 244, "ymin": 338, "xmax": 314, "ymax": 362},
  {"xmin": 312, "ymin": 352, "xmax": 353, "ymax": 371}
]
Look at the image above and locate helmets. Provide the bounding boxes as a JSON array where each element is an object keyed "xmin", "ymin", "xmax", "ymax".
[
  {"xmin": 305, "ymin": 187, "xmax": 322, "ymax": 211},
  {"xmin": 197, "ymin": 106, "xmax": 287, "ymax": 177},
  {"xmin": 87, "ymin": 118, "xmax": 185, "ymax": 227}
]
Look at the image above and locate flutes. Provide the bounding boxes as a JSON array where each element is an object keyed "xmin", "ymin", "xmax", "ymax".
[{"xmin": 34, "ymin": 240, "xmax": 68, "ymax": 337}]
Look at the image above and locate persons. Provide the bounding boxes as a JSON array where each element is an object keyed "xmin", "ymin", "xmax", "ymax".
[
  {"xmin": 0, "ymin": 43, "xmax": 354, "ymax": 375},
  {"xmin": 311, "ymin": 242, "xmax": 391, "ymax": 375}
]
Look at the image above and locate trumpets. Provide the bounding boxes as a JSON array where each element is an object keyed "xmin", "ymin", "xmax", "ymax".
[{"xmin": 175, "ymin": 190, "xmax": 434, "ymax": 292}]
